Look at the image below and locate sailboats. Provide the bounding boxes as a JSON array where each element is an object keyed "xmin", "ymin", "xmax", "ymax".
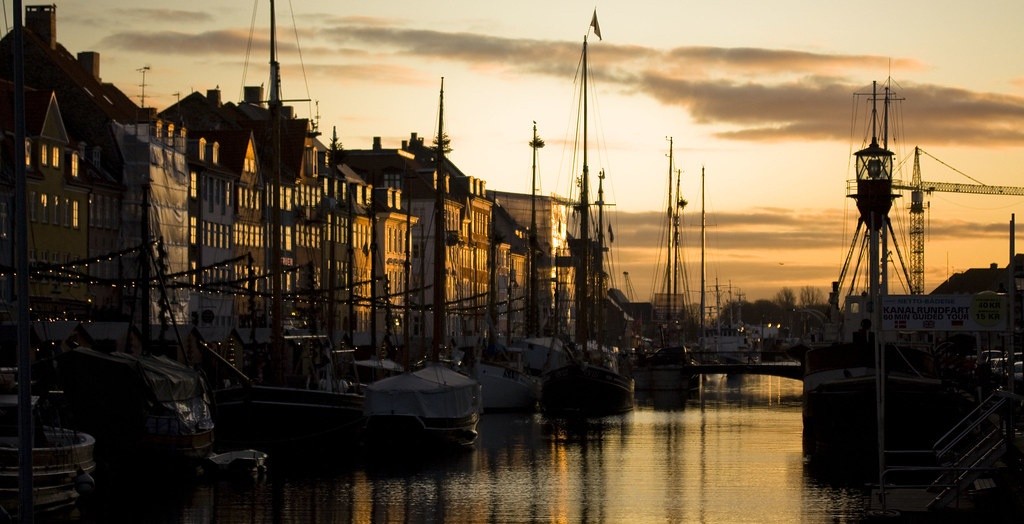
[
  {"xmin": 0, "ymin": 0, "xmax": 103, "ymax": 524},
  {"xmin": 179, "ymin": 0, "xmax": 711, "ymax": 453}
]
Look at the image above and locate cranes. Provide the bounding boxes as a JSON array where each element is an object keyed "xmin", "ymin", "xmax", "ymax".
[{"xmin": 846, "ymin": 148, "xmax": 1024, "ymax": 296}]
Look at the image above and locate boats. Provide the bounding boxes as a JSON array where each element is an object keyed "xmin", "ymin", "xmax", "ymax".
[
  {"xmin": 689, "ymin": 275, "xmax": 798, "ymax": 354},
  {"xmin": 54, "ymin": 174, "xmax": 218, "ymax": 484},
  {"xmin": 802, "ymin": 58, "xmax": 975, "ymax": 489}
]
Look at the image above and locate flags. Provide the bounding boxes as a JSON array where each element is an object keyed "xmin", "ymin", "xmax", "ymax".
[{"xmin": 591, "ymin": 10, "xmax": 602, "ymax": 40}]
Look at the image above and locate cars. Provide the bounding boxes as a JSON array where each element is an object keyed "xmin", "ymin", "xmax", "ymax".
[{"xmin": 983, "ymin": 350, "xmax": 1024, "ymax": 383}]
